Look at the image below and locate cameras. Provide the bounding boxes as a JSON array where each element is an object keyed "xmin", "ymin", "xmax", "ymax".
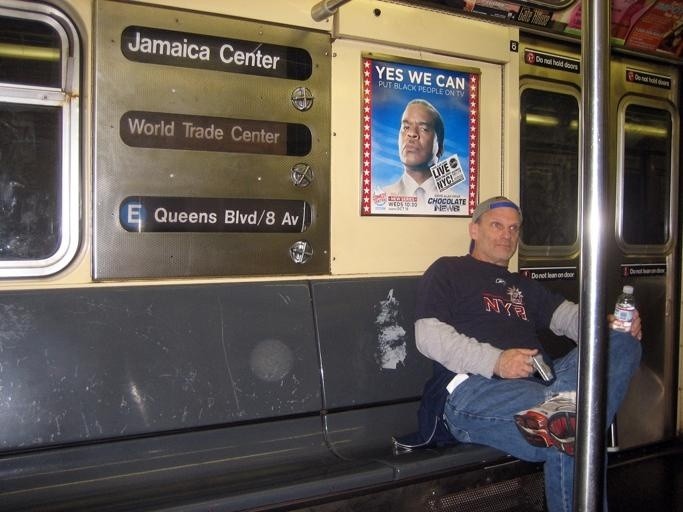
[{"xmin": 533, "ymin": 354, "xmax": 554, "ymax": 381}]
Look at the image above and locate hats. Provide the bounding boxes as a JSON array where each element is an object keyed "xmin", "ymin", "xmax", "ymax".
[{"xmin": 467, "ymin": 195, "xmax": 524, "ymax": 255}]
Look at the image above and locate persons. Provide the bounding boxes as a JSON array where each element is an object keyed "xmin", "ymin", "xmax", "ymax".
[
  {"xmin": 414, "ymin": 193, "xmax": 643, "ymax": 510},
  {"xmin": 377, "ymin": 95, "xmax": 459, "ymax": 210}
]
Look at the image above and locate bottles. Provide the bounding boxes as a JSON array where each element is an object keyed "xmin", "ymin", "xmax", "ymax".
[{"xmin": 611, "ymin": 286, "xmax": 635, "ymax": 333}]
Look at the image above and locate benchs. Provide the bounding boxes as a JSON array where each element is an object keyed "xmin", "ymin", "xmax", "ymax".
[
  {"xmin": 303, "ymin": 274, "xmax": 671, "ymax": 485},
  {"xmin": 0, "ymin": 273, "xmax": 396, "ymax": 512}
]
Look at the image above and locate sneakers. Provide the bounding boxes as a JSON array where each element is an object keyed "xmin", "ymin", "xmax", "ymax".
[{"xmin": 512, "ymin": 397, "xmax": 578, "ymax": 458}]
